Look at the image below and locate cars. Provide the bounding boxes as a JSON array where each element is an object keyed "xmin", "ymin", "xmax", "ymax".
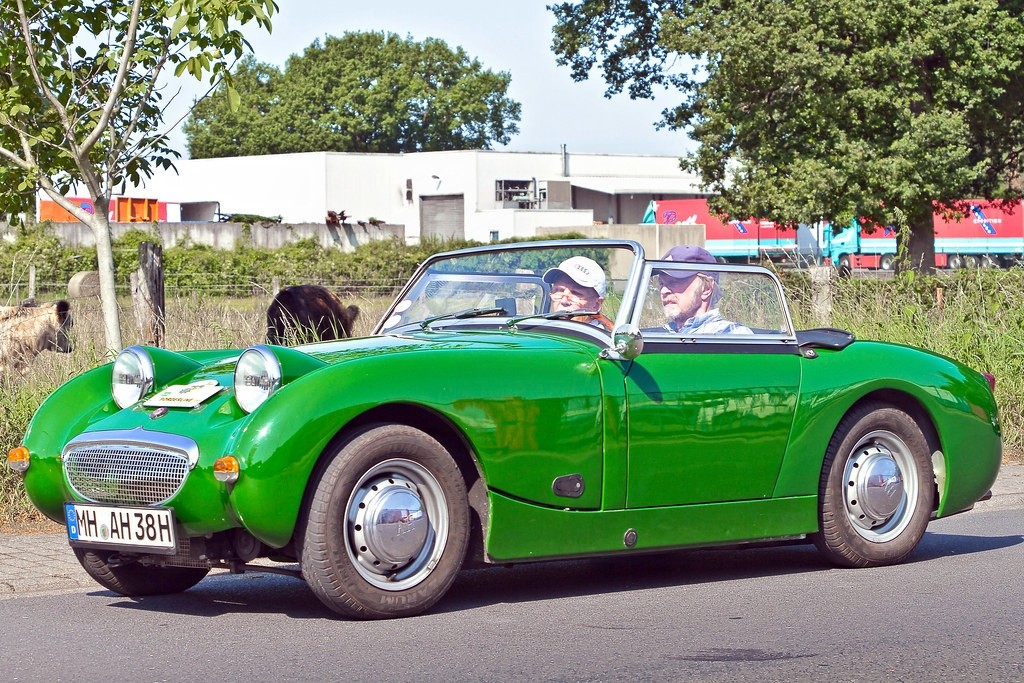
[{"xmin": 6, "ymin": 240, "xmax": 1003, "ymax": 620}]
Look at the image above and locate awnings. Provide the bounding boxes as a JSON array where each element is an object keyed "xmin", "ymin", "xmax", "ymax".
[{"xmin": 537, "ymin": 176, "xmax": 722, "ymax": 224}]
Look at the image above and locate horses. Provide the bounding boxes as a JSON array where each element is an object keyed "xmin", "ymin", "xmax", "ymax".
[
  {"xmin": 264, "ymin": 285, "xmax": 359, "ymax": 347},
  {"xmin": 0, "ymin": 298, "xmax": 77, "ymax": 443}
]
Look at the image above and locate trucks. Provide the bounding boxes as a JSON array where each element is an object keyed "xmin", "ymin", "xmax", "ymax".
[
  {"xmin": 824, "ymin": 197, "xmax": 1024, "ymax": 270},
  {"xmin": 642, "ymin": 199, "xmax": 823, "ymax": 262}
]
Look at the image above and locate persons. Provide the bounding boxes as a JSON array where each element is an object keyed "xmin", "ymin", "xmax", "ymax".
[
  {"xmin": 542, "ymin": 256, "xmax": 606, "ymax": 330},
  {"xmin": 651, "ymin": 245, "xmax": 755, "ymax": 334}
]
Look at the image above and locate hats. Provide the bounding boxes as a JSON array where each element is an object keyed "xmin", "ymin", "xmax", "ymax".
[
  {"xmin": 650, "ymin": 245, "xmax": 719, "ymax": 283},
  {"xmin": 542, "ymin": 256, "xmax": 607, "ymax": 297}
]
